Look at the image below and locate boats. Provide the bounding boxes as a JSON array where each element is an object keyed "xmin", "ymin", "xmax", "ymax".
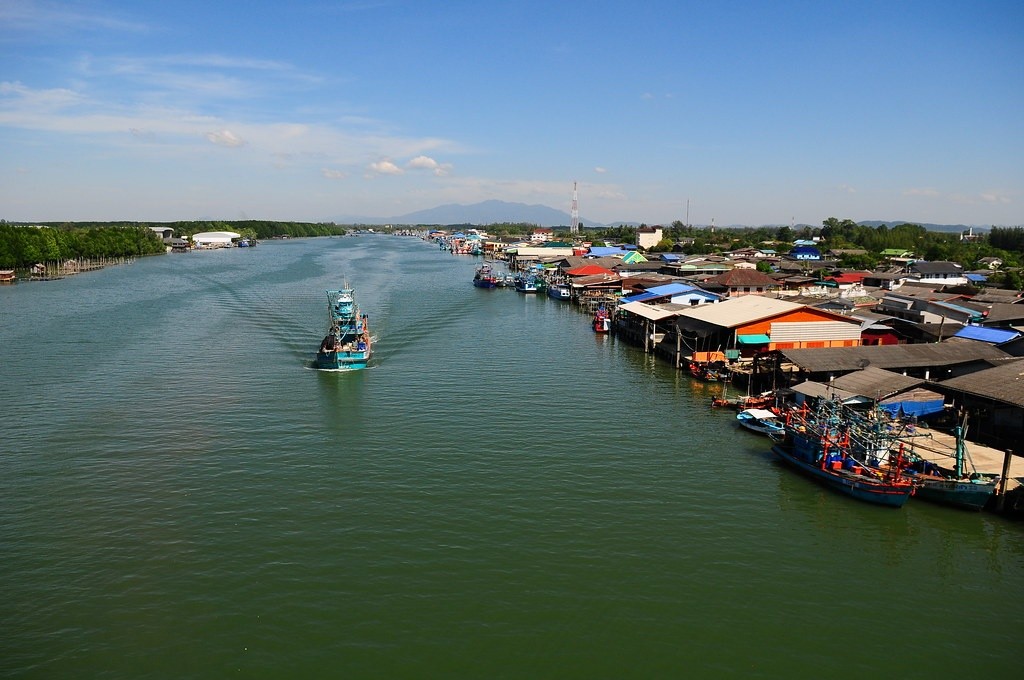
[
  {"xmin": 471, "ymin": 262, "xmax": 570, "ymax": 300},
  {"xmin": 688, "ymin": 362, "xmax": 719, "ymax": 382},
  {"xmin": 316, "ymin": 274, "xmax": 371, "ymax": 370},
  {"xmin": 712, "ymin": 376, "xmax": 999, "ymax": 511},
  {"xmin": 592, "ymin": 306, "xmax": 611, "ymax": 332}
]
